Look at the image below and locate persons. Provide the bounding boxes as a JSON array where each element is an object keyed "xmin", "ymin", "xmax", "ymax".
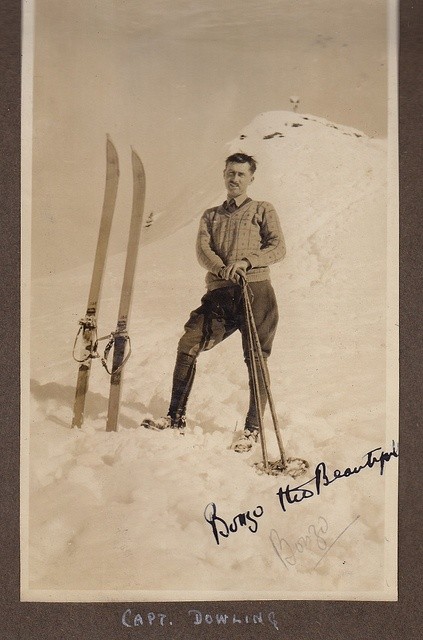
[{"xmin": 140, "ymin": 153, "xmax": 286, "ymax": 453}]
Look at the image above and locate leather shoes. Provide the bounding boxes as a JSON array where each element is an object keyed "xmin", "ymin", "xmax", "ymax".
[
  {"xmin": 140, "ymin": 415, "xmax": 186, "ymax": 432},
  {"xmin": 234, "ymin": 427, "xmax": 260, "ymax": 453}
]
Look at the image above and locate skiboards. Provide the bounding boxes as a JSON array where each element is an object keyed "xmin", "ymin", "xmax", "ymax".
[{"xmin": 71, "ymin": 137, "xmax": 146, "ymax": 431}]
